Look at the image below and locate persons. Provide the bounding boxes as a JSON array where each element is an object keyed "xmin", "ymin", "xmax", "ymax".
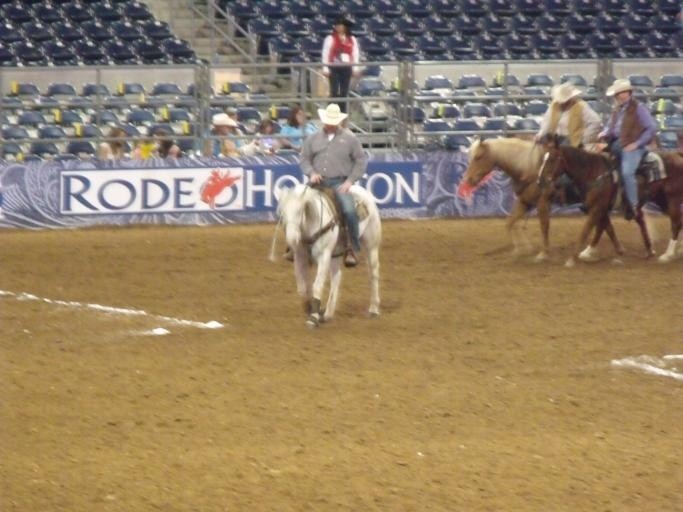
[
  {"xmin": 594, "ymin": 78, "xmax": 658, "ymax": 219},
  {"xmin": 95, "ymin": 100, "xmax": 318, "ymax": 160},
  {"xmin": 320, "ymin": 17, "xmax": 362, "ymax": 111},
  {"xmin": 532, "ymin": 82, "xmax": 605, "ymax": 217},
  {"xmin": 283, "ymin": 102, "xmax": 369, "ymax": 266}
]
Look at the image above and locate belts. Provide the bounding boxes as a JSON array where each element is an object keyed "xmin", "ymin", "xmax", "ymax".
[{"xmin": 322, "ymin": 175, "xmax": 348, "ymax": 181}]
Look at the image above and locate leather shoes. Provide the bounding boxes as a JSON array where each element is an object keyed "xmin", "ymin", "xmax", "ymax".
[{"xmin": 345, "ymin": 251, "xmax": 359, "ymax": 266}]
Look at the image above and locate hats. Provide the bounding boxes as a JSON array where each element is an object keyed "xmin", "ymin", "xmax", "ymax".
[
  {"xmin": 316, "ymin": 102, "xmax": 350, "ymax": 127},
  {"xmin": 211, "ymin": 112, "xmax": 238, "ymax": 127},
  {"xmin": 331, "ymin": 14, "xmax": 354, "ymax": 28},
  {"xmin": 552, "ymin": 81, "xmax": 583, "ymax": 105},
  {"xmin": 605, "ymin": 77, "xmax": 634, "ymax": 97}
]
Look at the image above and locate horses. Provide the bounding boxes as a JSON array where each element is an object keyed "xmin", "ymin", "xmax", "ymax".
[
  {"xmin": 276, "ymin": 183, "xmax": 383, "ymax": 327},
  {"xmin": 536, "ymin": 133, "xmax": 683, "ymax": 264},
  {"xmin": 463, "ymin": 133, "xmax": 625, "ymax": 267}
]
